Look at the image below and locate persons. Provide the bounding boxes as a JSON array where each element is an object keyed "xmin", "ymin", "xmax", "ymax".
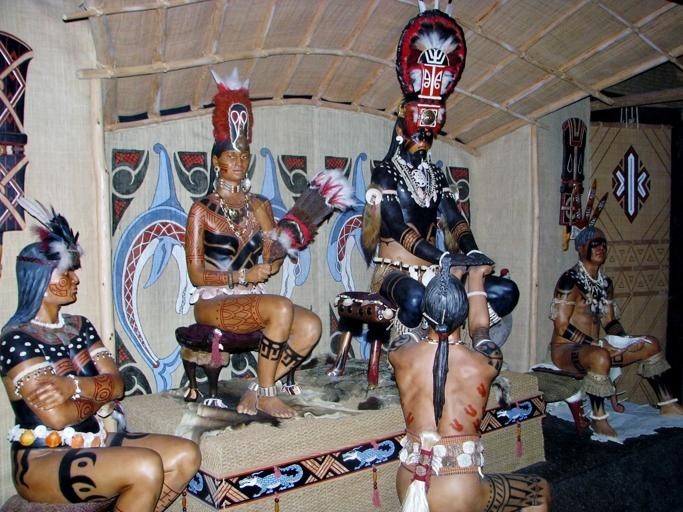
[
  {"xmin": 367, "ymin": 116, "xmax": 519, "ymax": 382},
  {"xmin": 0, "ymin": 199, "xmax": 202, "ymax": 511},
  {"xmin": 388, "ymin": 265, "xmax": 551, "ymax": 511},
  {"xmin": 184, "ymin": 141, "xmax": 322, "ymax": 419},
  {"xmin": 549, "ymin": 226, "xmax": 682, "ymax": 437}
]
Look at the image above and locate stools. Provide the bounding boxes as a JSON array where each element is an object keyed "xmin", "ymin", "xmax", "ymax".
[
  {"xmin": 175, "ymin": 322, "xmax": 300, "ymax": 409},
  {"xmin": 329, "ymin": 291, "xmax": 407, "ymax": 388},
  {"xmin": 530, "ymin": 364, "xmax": 655, "ymax": 434},
  {"xmin": 0, "ymin": 494, "xmax": 118, "ymax": 512}
]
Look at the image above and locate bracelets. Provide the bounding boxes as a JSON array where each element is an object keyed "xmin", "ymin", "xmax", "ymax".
[
  {"xmin": 439, "ymin": 251, "xmax": 450, "ymax": 268},
  {"xmin": 66, "ymin": 374, "xmax": 80, "ymax": 401},
  {"xmin": 228, "ymin": 270, "xmax": 234, "ymax": 289},
  {"xmin": 465, "ymin": 250, "xmax": 483, "ymax": 256},
  {"xmin": 238, "ymin": 268, "xmax": 247, "ymax": 286},
  {"xmin": 466, "ymin": 291, "xmax": 487, "ymax": 298},
  {"xmin": 598, "ymin": 339, "xmax": 603, "ymax": 349}
]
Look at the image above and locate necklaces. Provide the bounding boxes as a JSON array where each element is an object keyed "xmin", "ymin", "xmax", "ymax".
[
  {"xmin": 578, "ymin": 261, "xmax": 600, "ymax": 283},
  {"xmin": 218, "ymin": 178, "xmax": 241, "ymax": 193},
  {"xmin": 391, "ymin": 154, "xmax": 435, "ymax": 208},
  {"xmin": 30, "ymin": 311, "xmax": 65, "ymax": 330},
  {"xmin": 422, "ymin": 335, "xmax": 464, "ymax": 345},
  {"xmin": 574, "ymin": 264, "xmax": 607, "ymax": 318},
  {"xmin": 214, "ymin": 191, "xmax": 248, "ymax": 238}
]
